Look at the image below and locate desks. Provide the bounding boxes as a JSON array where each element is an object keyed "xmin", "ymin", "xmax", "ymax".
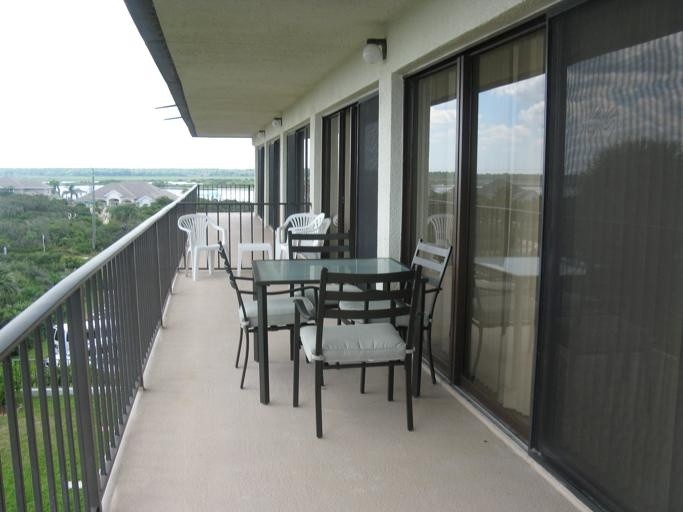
[
  {"xmin": 236, "ymin": 243, "xmax": 273, "ymax": 276},
  {"xmin": 251, "ymin": 257, "xmax": 423, "ymax": 403},
  {"xmin": 473, "ymin": 253, "xmax": 588, "ymax": 352}
]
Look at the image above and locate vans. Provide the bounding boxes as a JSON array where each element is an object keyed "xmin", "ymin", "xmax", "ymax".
[{"xmin": 52, "ymin": 319, "xmax": 126, "ymax": 358}]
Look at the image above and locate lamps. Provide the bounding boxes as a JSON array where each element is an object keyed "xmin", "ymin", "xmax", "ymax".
[
  {"xmin": 362, "ymin": 38, "xmax": 387, "ymax": 64},
  {"xmin": 258, "ymin": 130, "xmax": 265, "ymax": 138},
  {"xmin": 272, "ymin": 117, "xmax": 282, "ymax": 128}
]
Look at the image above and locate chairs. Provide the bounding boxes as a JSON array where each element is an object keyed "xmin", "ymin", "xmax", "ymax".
[
  {"xmin": 216, "ymin": 241, "xmax": 323, "ymax": 391},
  {"xmin": 285, "ymin": 214, "xmax": 331, "ymax": 261},
  {"xmin": 295, "ymin": 265, "xmax": 421, "ymax": 437},
  {"xmin": 338, "ymin": 239, "xmax": 451, "ymax": 393},
  {"xmin": 275, "ymin": 214, "xmax": 324, "ymax": 262},
  {"xmin": 473, "ymin": 277, "xmax": 533, "ymax": 372},
  {"xmin": 177, "ymin": 213, "xmax": 226, "ymax": 281},
  {"xmin": 284, "ymin": 227, "xmax": 366, "ymax": 361}
]
